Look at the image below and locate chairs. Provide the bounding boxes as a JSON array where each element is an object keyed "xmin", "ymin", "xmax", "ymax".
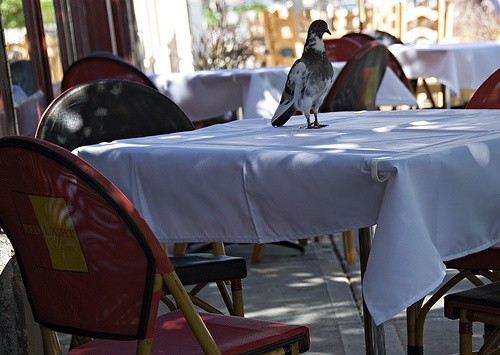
[{"xmin": 0, "ymin": 32, "xmax": 500, "ymax": 355}]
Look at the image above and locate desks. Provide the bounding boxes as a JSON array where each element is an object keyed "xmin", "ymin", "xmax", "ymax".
[
  {"xmin": 152, "ymin": 64, "xmax": 384, "ymax": 103},
  {"xmin": 387, "ymin": 42, "xmax": 500, "ymax": 106},
  {"xmin": 85, "ymin": 113, "xmax": 500, "ymax": 355}
]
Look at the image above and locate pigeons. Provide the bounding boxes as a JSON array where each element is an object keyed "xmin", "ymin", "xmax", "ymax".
[{"xmin": 272, "ymin": 19, "xmax": 335, "ymax": 129}]
